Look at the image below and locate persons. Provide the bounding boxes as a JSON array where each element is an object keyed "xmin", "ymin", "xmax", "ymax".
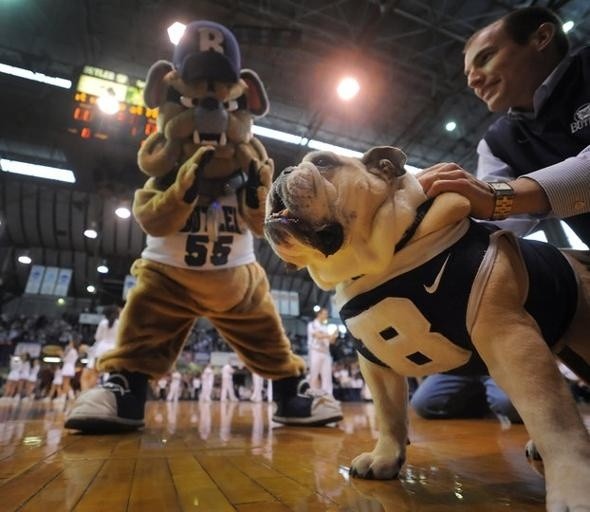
[
  {"xmin": 159, "ymin": 360, "xmax": 264, "ymax": 403},
  {"xmin": 0, "ymin": 303, "xmax": 120, "ymax": 404},
  {"xmin": 331, "ymin": 362, "xmax": 364, "ymax": 388},
  {"xmin": 407, "ymin": 3, "xmax": 590, "ymax": 420},
  {"xmin": 306, "ymin": 308, "xmax": 338, "ymax": 395}
]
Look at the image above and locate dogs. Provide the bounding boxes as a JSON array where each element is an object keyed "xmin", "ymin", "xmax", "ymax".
[{"xmin": 263, "ymin": 146, "xmax": 590, "ymax": 512}]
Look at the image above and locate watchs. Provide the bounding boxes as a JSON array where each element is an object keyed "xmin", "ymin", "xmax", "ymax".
[{"xmin": 486, "ymin": 180, "xmax": 516, "ymax": 221}]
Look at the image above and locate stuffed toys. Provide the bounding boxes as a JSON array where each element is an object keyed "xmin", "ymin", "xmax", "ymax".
[{"xmin": 57, "ymin": 16, "xmax": 348, "ymax": 435}]
